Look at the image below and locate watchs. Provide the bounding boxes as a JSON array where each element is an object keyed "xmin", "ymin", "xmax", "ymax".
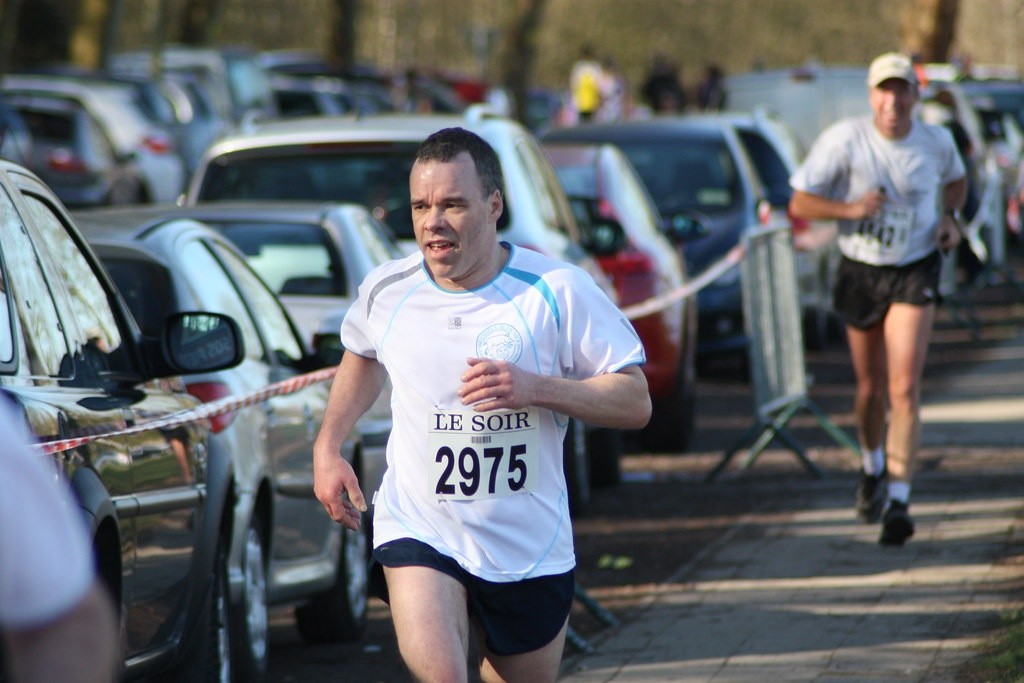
[{"xmin": 945, "ymin": 207, "xmax": 961, "ymax": 222}]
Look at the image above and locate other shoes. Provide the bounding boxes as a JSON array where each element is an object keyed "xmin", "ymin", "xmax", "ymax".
[
  {"xmin": 859, "ymin": 467, "xmax": 886, "ymax": 512},
  {"xmin": 879, "ymin": 500, "xmax": 914, "ymax": 546}
]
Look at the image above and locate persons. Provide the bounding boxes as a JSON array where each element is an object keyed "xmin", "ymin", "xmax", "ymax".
[
  {"xmin": 58, "ymin": 325, "xmax": 196, "ymax": 485},
  {"xmin": 0, "ymin": 392, "xmax": 123, "ymax": 683},
  {"xmin": 571, "ymin": 42, "xmax": 729, "ymax": 123},
  {"xmin": 312, "ymin": 128, "xmax": 649, "ymax": 683},
  {"xmin": 790, "ymin": 52, "xmax": 970, "ymax": 545}
]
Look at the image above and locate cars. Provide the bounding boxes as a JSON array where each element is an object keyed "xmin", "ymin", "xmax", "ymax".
[
  {"xmin": 1, "ymin": 156, "xmax": 248, "ymax": 683},
  {"xmin": 0, "ymin": 44, "xmax": 1024, "ymax": 591},
  {"xmin": 67, "ymin": 203, "xmax": 376, "ymax": 682}
]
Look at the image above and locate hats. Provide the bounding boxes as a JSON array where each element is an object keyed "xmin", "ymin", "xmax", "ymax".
[{"xmin": 869, "ymin": 51, "xmax": 918, "ymax": 88}]
